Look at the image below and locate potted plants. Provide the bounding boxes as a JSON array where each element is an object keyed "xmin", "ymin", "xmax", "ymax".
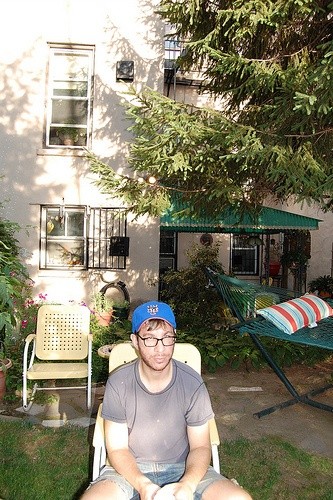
[
  {"xmin": 307, "ymin": 275, "xmax": 333, "ymax": 297},
  {"xmin": 0, "ymin": 277, "xmax": 20, "ymax": 403},
  {"xmin": 263, "ymin": 244, "xmax": 282, "ymax": 276},
  {"xmin": 279, "ymin": 245, "xmax": 311, "ymax": 283}
]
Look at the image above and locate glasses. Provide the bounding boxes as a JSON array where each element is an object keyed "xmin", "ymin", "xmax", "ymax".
[{"xmin": 137, "ymin": 332, "xmax": 176, "ymax": 347}]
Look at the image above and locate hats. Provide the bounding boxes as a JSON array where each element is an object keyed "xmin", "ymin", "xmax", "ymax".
[{"xmin": 132, "ymin": 301, "xmax": 177, "ymax": 333}]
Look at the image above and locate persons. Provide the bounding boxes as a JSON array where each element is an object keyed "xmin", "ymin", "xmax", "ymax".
[{"xmin": 80, "ymin": 301, "xmax": 252, "ymax": 500}]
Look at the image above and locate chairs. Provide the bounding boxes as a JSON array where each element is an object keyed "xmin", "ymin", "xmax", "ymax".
[
  {"xmin": 22, "ymin": 304, "xmax": 94, "ymax": 411},
  {"xmin": 92, "ymin": 342, "xmax": 220, "ymax": 480}
]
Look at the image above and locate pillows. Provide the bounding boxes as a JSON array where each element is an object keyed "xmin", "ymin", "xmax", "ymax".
[{"xmin": 255, "ymin": 292, "xmax": 333, "ymax": 335}]
